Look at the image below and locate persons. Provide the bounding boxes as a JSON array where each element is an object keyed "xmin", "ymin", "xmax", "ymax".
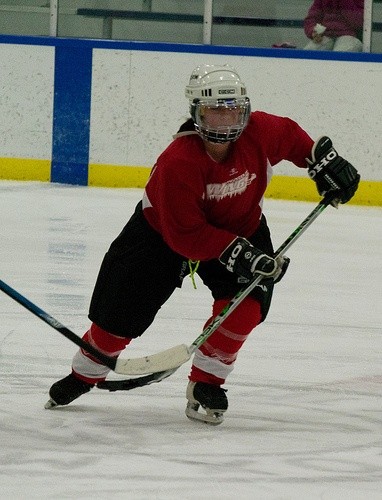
[
  {"xmin": 44, "ymin": 61, "xmax": 361, "ymax": 426},
  {"xmin": 302, "ymin": 0, "xmax": 364, "ymax": 53}
]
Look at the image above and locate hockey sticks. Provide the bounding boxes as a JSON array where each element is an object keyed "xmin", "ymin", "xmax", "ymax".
[
  {"xmin": 0, "ymin": 277, "xmax": 191, "ymax": 376},
  {"xmin": 91, "ymin": 192, "xmax": 329, "ymax": 392}
]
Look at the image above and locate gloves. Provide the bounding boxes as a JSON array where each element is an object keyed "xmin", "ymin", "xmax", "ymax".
[
  {"xmin": 306, "ymin": 136, "xmax": 360, "ymax": 208},
  {"xmin": 219, "ymin": 236, "xmax": 290, "ymax": 288}
]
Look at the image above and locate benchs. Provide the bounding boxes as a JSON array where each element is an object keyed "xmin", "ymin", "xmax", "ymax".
[{"xmin": 0, "ymin": 0, "xmax": 382, "ymax": 53}]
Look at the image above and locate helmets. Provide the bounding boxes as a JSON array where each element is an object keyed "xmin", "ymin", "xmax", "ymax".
[{"xmin": 186, "ymin": 63, "xmax": 250, "ymax": 145}]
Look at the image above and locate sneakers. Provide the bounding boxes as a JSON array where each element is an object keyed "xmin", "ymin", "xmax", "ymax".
[
  {"xmin": 186, "ymin": 380, "xmax": 228, "ymax": 424},
  {"xmin": 44, "ymin": 373, "xmax": 95, "ymax": 409}
]
